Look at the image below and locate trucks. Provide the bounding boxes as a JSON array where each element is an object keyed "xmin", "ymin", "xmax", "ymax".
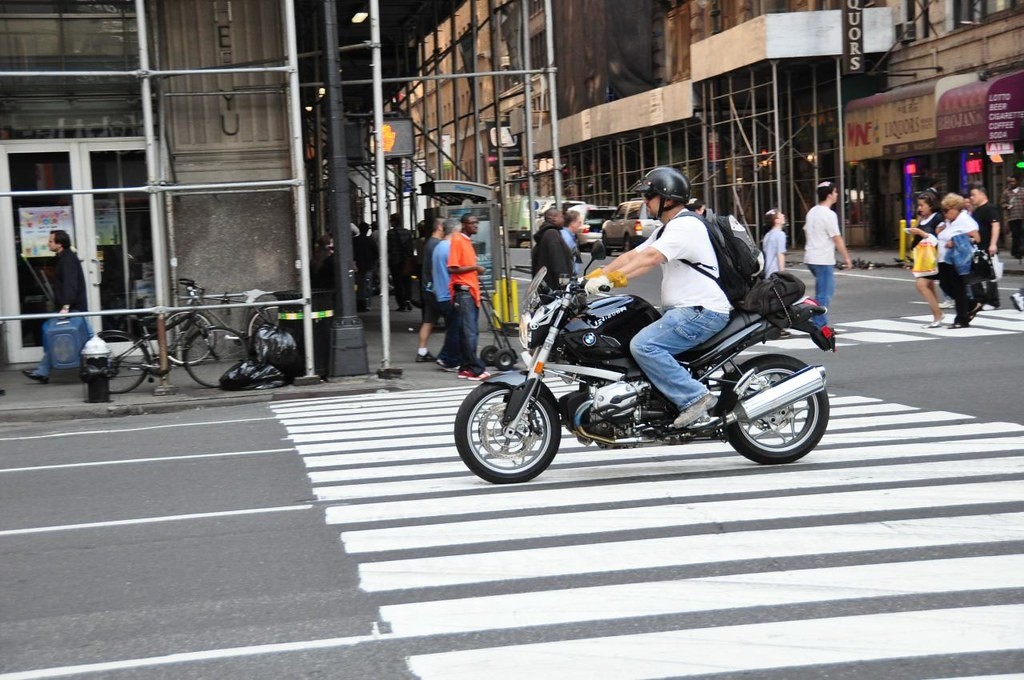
[{"xmin": 506, "ymin": 194, "xmax": 567, "ymax": 248}]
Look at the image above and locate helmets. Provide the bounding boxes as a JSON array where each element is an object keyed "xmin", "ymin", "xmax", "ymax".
[{"xmin": 634, "ymin": 167, "xmax": 690, "ymax": 205}]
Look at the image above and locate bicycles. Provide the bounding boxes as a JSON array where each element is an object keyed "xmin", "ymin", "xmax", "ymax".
[{"xmin": 80, "ymin": 278, "xmax": 279, "ymax": 388}]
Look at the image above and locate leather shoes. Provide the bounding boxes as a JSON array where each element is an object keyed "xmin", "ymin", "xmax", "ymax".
[{"xmin": 22, "ymin": 369, "xmax": 49, "ymax": 385}]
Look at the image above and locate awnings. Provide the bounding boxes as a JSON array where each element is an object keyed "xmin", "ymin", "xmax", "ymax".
[
  {"xmin": 844, "ymin": 72, "xmax": 978, "ymax": 162},
  {"xmin": 936, "ymin": 69, "xmax": 1024, "ymax": 148}
]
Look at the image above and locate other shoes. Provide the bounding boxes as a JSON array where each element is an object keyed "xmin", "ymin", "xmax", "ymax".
[
  {"xmin": 948, "ymin": 322, "xmax": 969, "ymax": 329},
  {"xmin": 938, "ymin": 299, "xmax": 956, "ymax": 310},
  {"xmin": 968, "ymin": 302, "xmax": 982, "ymax": 323},
  {"xmin": 415, "ymin": 352, "xmax": 436, "ymax": 362},
  {"xmin": 395, "ymin": 304, "xmax": 413, "ymax": 312},
  {"xmin": 926, "ymin": 312, "xmax": 945, "ymax": 328},
  {"xmin": 780, "ymin": 330, "xmax": 792, "ymax": 338},
  {"xmin": 436, "ymin": 358, "xmax": 461, "ymax": 373},
  {"xmin": 982, "ymin": 304, "xmax": 996, "ymax": 311},
  {"xmin": 1014, "ymin": 253, "xmax": 1022, "ymax": 259}
]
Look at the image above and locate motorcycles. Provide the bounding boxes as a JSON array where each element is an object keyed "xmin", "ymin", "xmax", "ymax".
[{"xmin": 454, "ymin": 239, "xmax": 838, "ymax": 484}]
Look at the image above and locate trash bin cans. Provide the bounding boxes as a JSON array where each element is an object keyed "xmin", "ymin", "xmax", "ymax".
[{"xmin": 272, "ymin": 289, "xmax": 332, "ymax": 384}]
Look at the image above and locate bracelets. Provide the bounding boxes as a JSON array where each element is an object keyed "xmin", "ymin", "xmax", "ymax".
[{"xmin": 63, "ymin": 305, "xmax": 69, "ymax": 309}]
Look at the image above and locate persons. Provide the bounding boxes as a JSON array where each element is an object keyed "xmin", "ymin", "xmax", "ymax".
[
  {"xmin": 909, "ymin": 192, "xmax": 945, "ymax": 328},
  {"xmin": 446, "ymin": 213, "xmax": 490, "ymax": 381},
  {"xmin": 352, "ymin": 213, "xmax": 425, "ymax": 312},
  {"xmin": 1000, "ymin": 177, "xmax": 1024, "ymax": 258},
  {"xmin": 936, "ymin": 193, "xmax": 982, "ymax": 328},
  {"xmin": 1010, "ymin": 288, "xmax": 1024, "ymax": 312},
  {"xmin": 964, "ymin": 186, "xmax": 1001, "ymax": 311},
  {"xmin": 415, "ymin": 215, "xmax": 446, "ymax": 362},
  {"xmin": 431, "ymin": 216, "xmax": 463, "ymax": 372},
  {"xmin": 802, "ymin": 181, "xmax": 851, "ymax": 326},
  {"xmin": 560, "ymin": 210, "xmax": 581, "ymax": 273},
  {"xmin": 22, "ymin": 230, "xmax": 88, "ymax": 385},
  {"xmin": 764, "ymin": 210, "xmax": 791, "ymax": 337},
  {"xmin": 579, "ymin": 167, "xmax": 730, "ymax": 428},
  {"xmin": 530, "ymin": 208, "xmax": 574, "ymax": 297}
]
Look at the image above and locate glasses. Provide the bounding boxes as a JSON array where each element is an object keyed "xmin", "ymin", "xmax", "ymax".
[
  {"xmin": 940, "ymin": 207, "xmax": 953, "ymax": 212},
  {"xmin": 644, "ymin": 188, "xmax": 659, "ymax": 202},
  {"xmin": 461, "ymin": 221, "xmax": 479, "ymax": 225}
]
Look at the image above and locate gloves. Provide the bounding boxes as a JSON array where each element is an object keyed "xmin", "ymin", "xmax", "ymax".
[
  {"xmin": 565, "ymin": 268, "xmax": 603, "ymax": 293},
  {"xmin": 584, "ymin": 270, "xmax": 628, "ymax": 297}
]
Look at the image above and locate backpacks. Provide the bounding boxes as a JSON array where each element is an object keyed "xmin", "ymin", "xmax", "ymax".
[{"xmin": 663, "ymin": 210, "xmax": 766, "ymax": 308}]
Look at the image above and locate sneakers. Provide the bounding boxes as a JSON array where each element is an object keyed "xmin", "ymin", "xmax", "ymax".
[
  {"xmin": 1011, "ymin": 292, "xmax": 1024, "ymax": 311},
  {"xmin": 457, "ymin": 370, "xmax": 491, "ymax": 380},
  {"xmin": 673, "ymin": 392, "xmax": 719, "ymax": 428}
]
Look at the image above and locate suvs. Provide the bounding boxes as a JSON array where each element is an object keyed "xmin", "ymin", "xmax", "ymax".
[
  {"xmin": 541, "ymin": 201, "xmax": 617, "ymax": 251},
  {"xmin": 602, "ymin": 198, "xmax": 664, "ymax": 253}
]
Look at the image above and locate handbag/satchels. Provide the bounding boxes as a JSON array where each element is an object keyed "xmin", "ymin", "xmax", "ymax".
[
  {"xmin": 961, "ymin": 248, "xmax": 996, "ymax": 287},
  {"xmin": 989, "ymin": 254, "xmax": 1004, "ymax": 283},
  {"xmin": 912, "ymin": 234, "xmax": 939, "ymax": 278},
  {"xmin": 398, "ymin": 255, "xmax": 417, "ymax": 277}
]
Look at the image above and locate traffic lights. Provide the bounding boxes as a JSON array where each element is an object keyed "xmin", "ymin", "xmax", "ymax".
[{"xmin": 383, "ymin": 117, "xmax": 415, "ymax": 157}]
